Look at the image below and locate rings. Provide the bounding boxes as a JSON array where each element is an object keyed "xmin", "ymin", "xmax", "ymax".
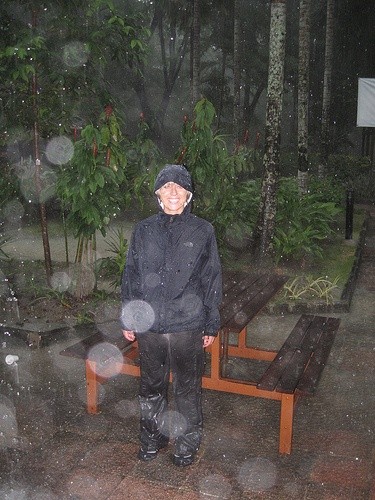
[{"xmin": 212, "ymin": 339, "xmax": 214, "ymax": 343}]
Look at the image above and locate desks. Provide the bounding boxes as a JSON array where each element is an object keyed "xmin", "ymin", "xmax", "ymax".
[{"xmin": 203, "ymin": 270, "xmax": 289, "ymax": 379}]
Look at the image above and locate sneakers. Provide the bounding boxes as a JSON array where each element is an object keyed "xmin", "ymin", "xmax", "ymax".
[
  {"xmin": 173, "ymin": 441, "xmax": 197, "ymax": 466},
  {"xmin": 138, "ymin": 436, "xmax": 169, "ymax": 460}
]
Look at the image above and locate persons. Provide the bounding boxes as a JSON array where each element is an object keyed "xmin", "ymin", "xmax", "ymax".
[{"xmin": 119, "ymin": 164, "xmax": 222, "ymax": 466}]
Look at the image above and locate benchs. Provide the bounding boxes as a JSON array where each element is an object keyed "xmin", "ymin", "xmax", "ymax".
[
  {"xmin": 256, "ymin": 314, "xmax": 341, "ymax": 455},
  {"xmin": 59, "ymin": 322, "xmax": 136, "ymax": 413}
]
